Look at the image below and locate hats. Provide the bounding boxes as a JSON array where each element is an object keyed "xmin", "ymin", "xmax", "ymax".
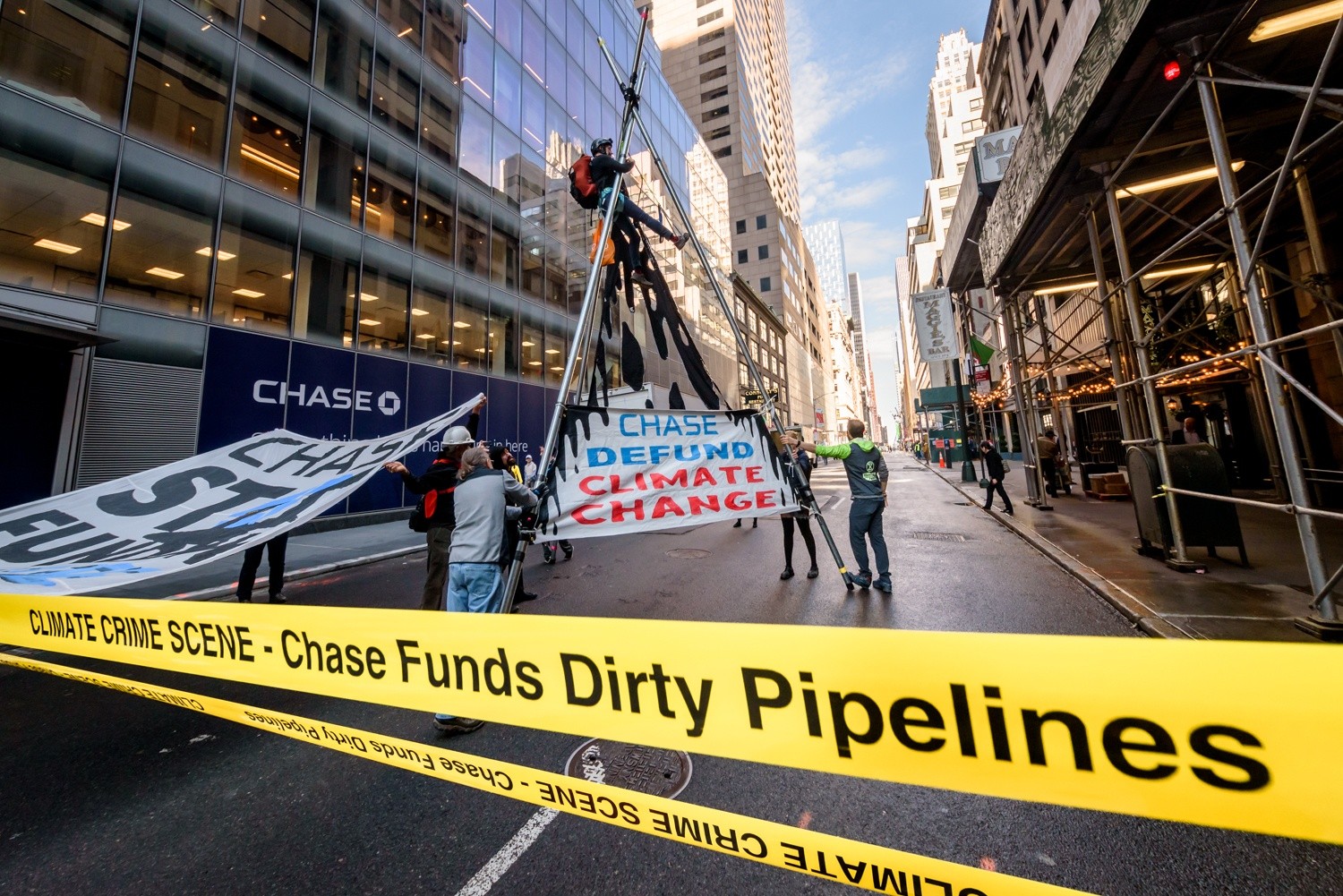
[
  {"xmin": 1045, "ymin": 430, "xmax": 1057, "ymax": 438},
  {"xmin": 526, "ymin": 454, "xmax": 532, "ymax": 459}
]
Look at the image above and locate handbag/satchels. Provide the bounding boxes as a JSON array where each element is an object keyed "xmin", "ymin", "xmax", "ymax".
[
  {"xmin": 408, "ymin": 497, "xmax": 427, "ymax": 533},
  {"xmin": 589, "ymin": 220, "xmax": 615, "ymax": 265}
]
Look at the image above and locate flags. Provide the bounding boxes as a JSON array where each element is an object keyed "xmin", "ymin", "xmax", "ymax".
[{"xmin": 963, "ymin": 331, "xmax": 995, "ymax": 375}]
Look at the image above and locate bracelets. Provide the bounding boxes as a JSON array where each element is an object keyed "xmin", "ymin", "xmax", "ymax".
[{"xmin": 796, "ymin": 440, "xmax": 801, "ymax": 448}]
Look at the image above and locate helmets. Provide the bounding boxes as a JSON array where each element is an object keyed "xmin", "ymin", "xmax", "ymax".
[
  {"xmin": 590, "ymin": 137, "xmax": 613, "ymax": 155},
  {"xmin": 441, "ymin": 426, "xmax": 476, "ymax": 446}
]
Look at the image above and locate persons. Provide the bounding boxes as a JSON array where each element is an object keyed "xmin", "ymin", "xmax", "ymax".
[
  {"xmin": 1172, "ymin": 417, "xmax": 1204, "ymax": 444},
  {"xmin": 922, "ymin": 440, "xmax": 929, "ymax": 465},
  {"xmin": 235, "ymin": 531, "xmax": 288, "ymax": 603},
  {"xmin": 915, "ymin": 442, "xmax": 921, "ymax": 460},
  {"xmin": 979, "ymin": 440, "xmax": 1013, "ymax": 514},
  {"xmin": 733, "ymin": 517, "xmax": 757, "ymax": 528},
  {"xmin": 823, "ymin": 457, "xmax": 827, "ymax": 466},
  {"xmin": 589, "ymin": 137, "xmax": 690, "ymax": 288},
  {"xmin": 779, "ymin": 431, "xmax": 818, "ymax": 579},
  {"xmin": 1030, "ymin": 430, "xmax": 1072, "ymax": 497},
  {"xmin": 780, "ymin": 419, "xmax": 892, "ymax": 592},
  {"xmin": 384, "ymin": 393, "xmax": 575, "ymax": 614}
]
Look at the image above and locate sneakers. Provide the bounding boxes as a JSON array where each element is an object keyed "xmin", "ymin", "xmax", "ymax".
[
  {"xmin": 435, "ymin": 716, "xmax": 486, "ymax": 731},
  {"xmin": 845, "ymin": 569, "xmax": 872, "ymax": 589},
  {"xmin": 873, "ymin": 572, "xmax": 892, "ymax": 592}
]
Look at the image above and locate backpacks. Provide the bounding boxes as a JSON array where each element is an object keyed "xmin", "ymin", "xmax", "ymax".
[
  {"xmin": 1001, "ymin": 459, "xmax": 1010, "ymax": 473},
  {"xmin": 567, "ymin": 153, "xmax": 599, "ymax": 210}
]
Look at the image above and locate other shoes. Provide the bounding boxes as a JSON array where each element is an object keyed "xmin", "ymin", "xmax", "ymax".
[
  {"xmin": 807, "ymin": 567, "xmax": 819, "ymax": 579},
  {"xmin": 269, "ymin": 593, "xmax": 286, "ymax": 604},
  {"xmin": 733, "ymin": 521, "xmax": 742, "ymax": 527},
  {"xmin": 512, "ymin": 592, "xmax": 537, "ymax": 603},
  {"xmin": 780, "ymin": 566, "xmax": 794, "ymax": 579},
  {"xmin": 753, "ymin": 522, "xmax": 758, "ymax": 528},
  {"xmin": 1001, "ymin": 509, "xmax": 1013, "ymax": 516},
  {"xmin": 562, "ymin": 545, "xmax": 574, "ymax": 561},
  {"xmin": 982, "ymin": 506, "xmax": 991, "ymax": 510}
]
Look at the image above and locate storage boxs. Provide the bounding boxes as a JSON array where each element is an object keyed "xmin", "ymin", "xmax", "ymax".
[{"xmin": 1088, "ymin": 471, "xmax": 1127, "ymax": 494}]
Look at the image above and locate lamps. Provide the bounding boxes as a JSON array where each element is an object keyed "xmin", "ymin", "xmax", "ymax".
[{"xmin": 1032, "ymin": 0, "xmax": 1343, "ymax": 298}]
